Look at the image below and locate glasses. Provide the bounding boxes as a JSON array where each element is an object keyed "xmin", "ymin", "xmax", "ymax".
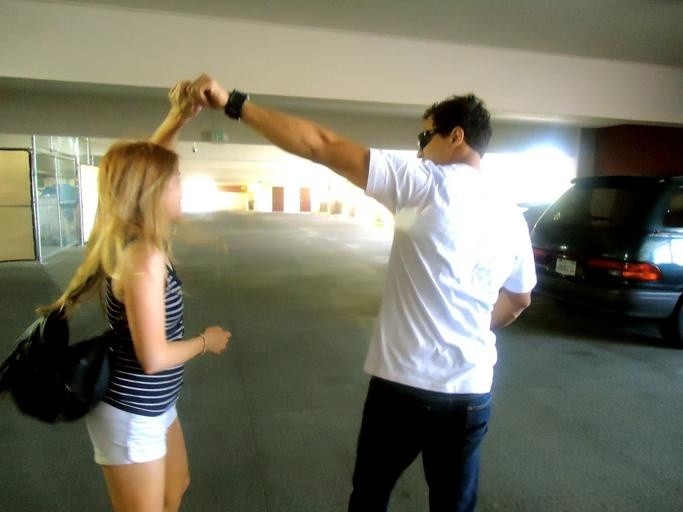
[{"xmin": 416, "ymin": 124, "xmax": 444, "ymax": 149}]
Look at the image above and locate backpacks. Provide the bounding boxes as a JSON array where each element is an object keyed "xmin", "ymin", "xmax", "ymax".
[{"xmin": 1, "ymin": 231, "xmax": 139, "ymax": 425}]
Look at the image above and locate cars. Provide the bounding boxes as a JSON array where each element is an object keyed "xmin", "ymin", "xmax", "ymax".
[{"xmin": 529, "ymin": 175, "xmax": 682, "ymax": 349}]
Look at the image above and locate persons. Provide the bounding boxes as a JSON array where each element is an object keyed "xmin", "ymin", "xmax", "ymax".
[
  {"xmin": 30, "ymin": 80, "xmax": 235, "ymax": 512},
  {"xmin": 184, "ymin": 72, "xmax": 540, "ymax": 509}
]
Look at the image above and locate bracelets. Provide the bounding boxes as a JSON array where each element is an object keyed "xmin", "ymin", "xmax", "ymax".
[
  {"xmin": 197, "ymin": 331, "xmax": 207, "ymax": 358},
  {"xmin": 224, "ymin": 87, "xmax": 248, "ymax": 121}
]
